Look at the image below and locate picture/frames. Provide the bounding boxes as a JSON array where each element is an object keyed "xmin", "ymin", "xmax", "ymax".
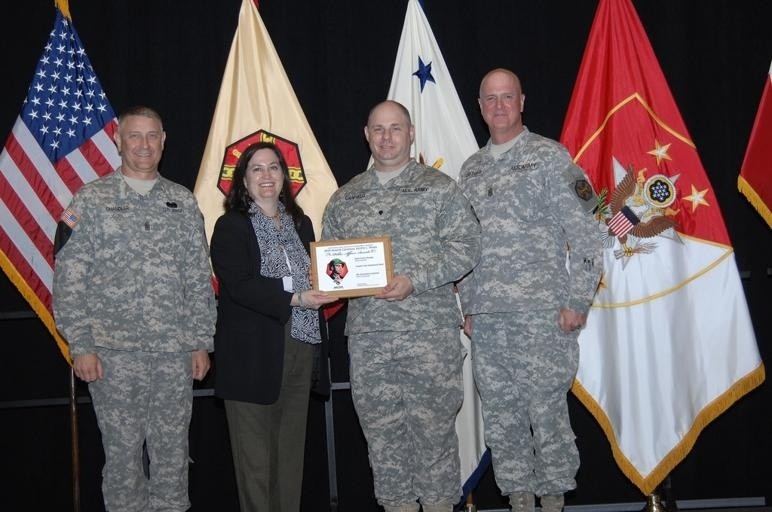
[{"xmin": 309, "ymin": 236, "xmax": 394, "ymax": 300}]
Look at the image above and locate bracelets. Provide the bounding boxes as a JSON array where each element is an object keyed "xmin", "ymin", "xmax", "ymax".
[{"xmin": 298, "ymin": 290, "xmax": 306, "ymax": 312}]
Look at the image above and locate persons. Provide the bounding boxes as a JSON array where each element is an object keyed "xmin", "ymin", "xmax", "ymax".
[
  {"xmin": 210, "ymin": 142, "xmax": 338, "ymax": 509},
  {"xmin": 328, "ymin": 258, "xmax": 345, "ymax": 284},
  {"xmin": 456, "ymin": 70, "xmax": 605, "ymax": 511},
  {"xmin": 51, "ymin": 106, "xmax": 217, "ymax": 512},
  {"xmin": 321, "ymin": 99, "xmax": 482, "ymax": 511}
]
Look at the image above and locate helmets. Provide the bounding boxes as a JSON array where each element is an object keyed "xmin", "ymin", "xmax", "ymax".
[{"xmin": 329, "ymin": 259, "xmax": 345, "ymax": 270}]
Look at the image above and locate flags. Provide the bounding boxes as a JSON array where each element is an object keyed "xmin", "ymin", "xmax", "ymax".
[
  {"xmin": 0, "ymin": 2, "xmax": 123, "ymax": 370},
  {"xmin": 737, "ymin": 60, "xmax": 772, "ymax": 230},
  {"xmin": 365, "ymin": 0, "xmax": 493, "ymax": 502},
  {"xmin": 191, "ymin": 1, "xmax": 348, "ymax": 323},
  {"xmin": 560, "ymin": 2, "xmax": 765, "ymax": 495}
]
